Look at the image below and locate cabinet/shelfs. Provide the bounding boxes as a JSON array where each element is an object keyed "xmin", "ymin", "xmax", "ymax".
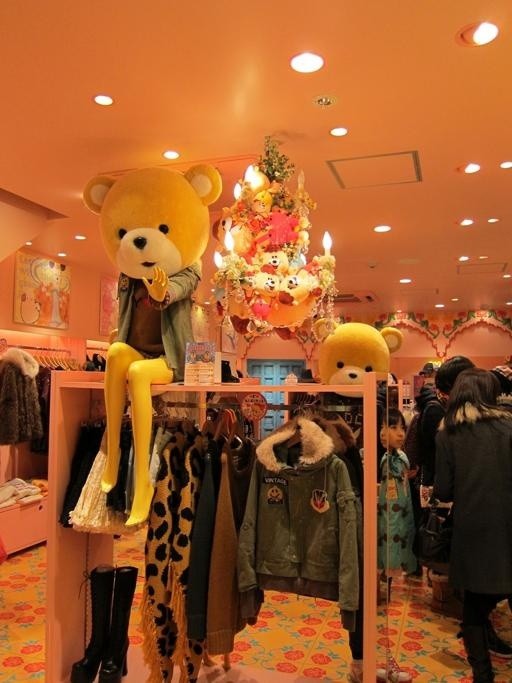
[
  {"xmin": 210, "ymin": 376, "xmax": 263, "ymax": 435},
  {"xmin": 0, "ymin": 490, "xmax": 49, "ymax": 557}
]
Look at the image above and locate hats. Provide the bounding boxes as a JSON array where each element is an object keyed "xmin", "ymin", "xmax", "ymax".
[
  {"xmin": 488, "ymin": 364, "xmax": 511, "ymax": 381},
  {"xmin": 435, "ymin": 355, "xmax": 474, "ymax": 392}
]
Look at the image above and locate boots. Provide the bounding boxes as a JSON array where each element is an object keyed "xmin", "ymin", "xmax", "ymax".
[
  {"xmin": 460, "ymin": 617, "xmax": 496, "ymax": 682},
  {"xmin": 99, "ymin": 565, "xmax": 139, "ymax": 681},
  {"xmin": 71, "ymin": 562, "xmax": 115, "ymax": 683}
]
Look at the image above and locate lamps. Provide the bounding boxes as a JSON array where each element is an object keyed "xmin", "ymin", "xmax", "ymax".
[{"xmin": 205, "ymin": 148, "xmax": 339, "ymax": 373}]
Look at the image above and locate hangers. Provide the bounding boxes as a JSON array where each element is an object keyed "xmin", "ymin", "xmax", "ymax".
[
  {"xmin": 92, "ymin": 403, "xmax": 325, "ymax": 463},
  {"xmin": 34, "ymin": 347, "xmax": 83, "ymax": 373}
]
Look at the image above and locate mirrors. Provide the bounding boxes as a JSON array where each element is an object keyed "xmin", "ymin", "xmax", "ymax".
[{"xmin": 376, "ymin": 378, "xmax": 390, "ymax": 682}]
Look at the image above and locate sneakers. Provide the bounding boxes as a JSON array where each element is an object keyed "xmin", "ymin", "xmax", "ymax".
[
  {"xmin": 348, "ymin": 650, "xmax": 412, "ymax": 682},
  {"xmin": 459, "ymin": 617, "xmax": 511, "ymax": 658}
]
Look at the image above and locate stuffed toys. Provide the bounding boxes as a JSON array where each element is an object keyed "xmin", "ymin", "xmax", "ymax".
[
  {"xmin": 247, "ymin": 263, "xmax": 281, "ymax": 322},
  {"xmin": 212, "ymin": 206, "xmax": 251, "ymax": 253},
  {"xmin": 312, "ymin": 316, "xmax": 405, "ymax": 399},
  {"xmin": 260, "ymin": 242, "xmax": 289, "ymax": 278},
  {"xmin": 238, "ymin": 163, "xmax": 282, "ymax": 220},
  {"xmin": 278, "ymin": 253, "xmax": 320, "ymax": 307}
]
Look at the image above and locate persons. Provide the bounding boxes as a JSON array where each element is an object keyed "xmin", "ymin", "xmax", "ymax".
[
  {"xmin": 388, "ymin": 406, "xmax": 418, "ymax": 681},
  {"xmin": 433, "ymin": 368, "xmax": 512, "ymax": 682},
  {"xmin": 377, "ymin": 414, "xmax": 388, "ymax": 681},
  {"xmin": 81, "ymin": 163, "xmax": 224, "ymax": 526},
  {"xmin": 410, "ymin": 356, "xmax": 511, "ymax": 657}
]
